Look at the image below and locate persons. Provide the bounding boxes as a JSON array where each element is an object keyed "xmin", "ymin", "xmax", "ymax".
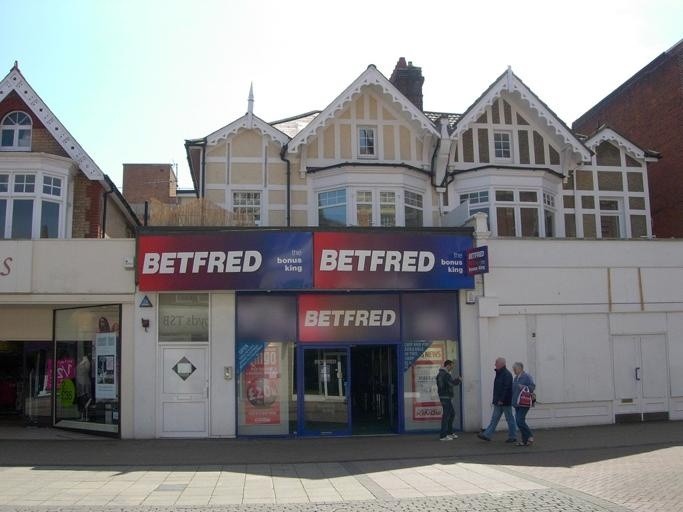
[
  {"xmin": 436, "ymin": 359, "xmax": 463, "ymax": 440},
  {"xmin": 512, "ymin": 361, "xmax": 536, "ymax": 446},
  {"xmin": 476, "ymin": 357, "xmax": 517, "ymax": 443},
  {"xmin": 76, "ymin": 355, "xmax": 91, "ymax": 400}
]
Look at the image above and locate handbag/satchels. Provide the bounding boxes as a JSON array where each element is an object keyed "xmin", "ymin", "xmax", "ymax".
[{"xmin": 517, "ymin": 386, "xmax": 532, "ymax": 408}]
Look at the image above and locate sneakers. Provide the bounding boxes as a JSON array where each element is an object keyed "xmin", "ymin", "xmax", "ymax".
[
  {"xmin": 440, "ymin": 434, "xmax": 459, "ymax": 440},
  {"xmin": 506, "ymin": 438, "xmax": 516, "ymax": 442},
  {"xmin": 479, "ymin": 434, "xmax": 491, "ymax": 441}
]
